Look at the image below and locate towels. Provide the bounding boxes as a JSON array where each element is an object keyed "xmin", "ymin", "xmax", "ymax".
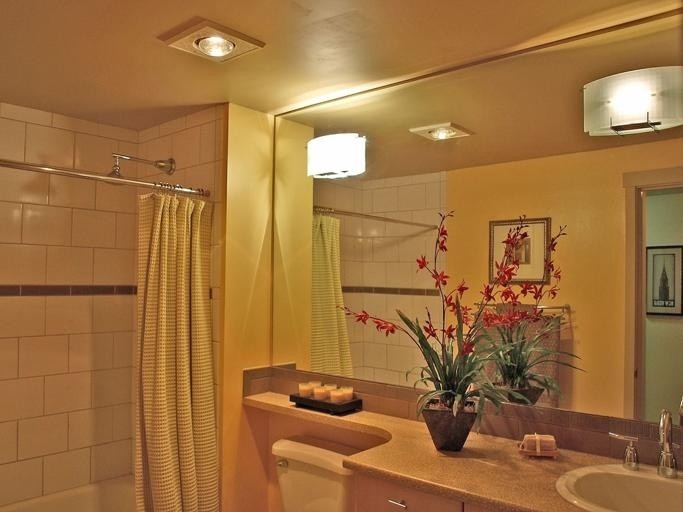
[{"xmin": 470, "ymin": 312, "xmax": 560, "ymax": 404}]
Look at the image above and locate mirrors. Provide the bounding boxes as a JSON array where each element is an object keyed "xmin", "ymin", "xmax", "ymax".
[{"xmin": 271, "ymin": 10, "xmax": 683, "ymax": 426}]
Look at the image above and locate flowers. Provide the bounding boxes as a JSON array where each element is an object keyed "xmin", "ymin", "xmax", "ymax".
[
  {"xmin": 412, "ymin": 212, "xmax": 590, "ymax": 403},
  {"xmin": 335, "ymin": 210, "xmax": 532, "ymax": 436}
]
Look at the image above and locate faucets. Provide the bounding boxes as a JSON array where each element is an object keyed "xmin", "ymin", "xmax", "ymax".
[
  {"xmin": 678, "ymin": 397, "xmax": 683, "ymax": 426},
  {"xmin": 657, "ymin": 409, "xmax": 677, "ymax": 479}
]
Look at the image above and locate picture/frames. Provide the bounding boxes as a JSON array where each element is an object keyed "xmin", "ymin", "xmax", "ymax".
[
  {"xmin": 487, "ymin": 217, "xmax": 551, "ymax": 285},
  {"xmin": 644, "ymin": 246, "xmax": 682, "ymax": 317}
]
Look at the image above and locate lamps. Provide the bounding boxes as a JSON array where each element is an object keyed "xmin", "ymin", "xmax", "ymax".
[
  {"xmin": 582, "ymin": 66, "xmax": 683, "ymax": 138},
  {"xmin": 305, "ymin": 133, "xmax": 365, "ymax": 181},
  {"xmin": 406, "ymin": 123, "xmax": 472, "ymax": 142},
  {"xmin": 163, "ymin": 19, "xmax": 264, "ymax": 65}
]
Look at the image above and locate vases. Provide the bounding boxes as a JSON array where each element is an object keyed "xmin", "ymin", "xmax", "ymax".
[
  {"xmin": 421, "ymin": 409, "xmax": 476, "ymax": 453},
  {"xmin": 491, "ymin": 383, "xmax": 543, "ymax": 406}
]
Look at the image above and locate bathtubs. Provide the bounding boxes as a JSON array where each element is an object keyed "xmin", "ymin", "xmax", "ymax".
[{"xmin": 1, "ymin": 473, "xmax": 136, "ymax": 512}]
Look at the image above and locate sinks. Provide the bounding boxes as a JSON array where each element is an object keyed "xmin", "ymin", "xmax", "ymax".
[{"xmin": 555, "ymin": 463, "xmax": 683, "ymax": 512}]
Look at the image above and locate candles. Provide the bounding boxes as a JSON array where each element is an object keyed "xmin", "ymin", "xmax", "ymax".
[{"xmin": 298, "ymin": 379, "xmax": 355, "ymax": 406}]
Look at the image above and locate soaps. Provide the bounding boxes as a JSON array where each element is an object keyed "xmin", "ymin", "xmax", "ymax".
[{"xmin": 523, "ymin": 434, "xmax": 556, "ymax": 451}]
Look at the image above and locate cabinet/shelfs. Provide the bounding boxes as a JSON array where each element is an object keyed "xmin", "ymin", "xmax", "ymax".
[{"xmin": 353, "ymin": 469, "xmax": 594, "ymax": 511}]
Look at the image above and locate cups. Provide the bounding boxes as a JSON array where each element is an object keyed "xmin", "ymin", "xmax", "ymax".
[
  {"xmin": 309, "ymin": 380, "xmax": 353, "ymax": 404},
  {"xmin": 298, "ymin": 382, "xmax": 313, "ymax": 398}
]
